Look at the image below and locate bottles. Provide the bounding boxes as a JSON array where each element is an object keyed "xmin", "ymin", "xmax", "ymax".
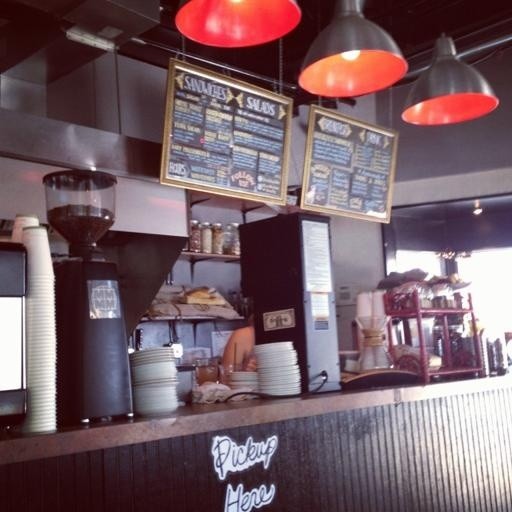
[
  {"xmin": 190, "ymin": 217, "xmax": 241, "ymax": 255},
  {"xmin": 355, "ymin": 315, "xmax": 395, "ymax": 376}
]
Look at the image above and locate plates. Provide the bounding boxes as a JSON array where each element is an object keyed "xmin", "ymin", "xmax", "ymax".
[
  {"xmin": 228, "ymin": 341, "xmax": 301, "ymax": 399},
  {"xmin": 129, "ymin": 345, "xmax": 184, "ymax": 417}
]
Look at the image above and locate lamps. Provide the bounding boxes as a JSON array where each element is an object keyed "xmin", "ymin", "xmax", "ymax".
[
  {"xmin": 174, "ymin": 0, "xmax": 301, "ymax": 49},
  {"xmin": 297, "ymin": 1, "xmax": 409, "ymax": 97},
  {"xmin": 401, "ymin": 32, "xmax": 499, "ymax": 126}
]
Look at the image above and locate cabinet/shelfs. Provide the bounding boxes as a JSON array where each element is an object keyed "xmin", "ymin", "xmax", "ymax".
[{"xmin": 383, "ymin": 289, "xmax": 485, "ymax": 384}]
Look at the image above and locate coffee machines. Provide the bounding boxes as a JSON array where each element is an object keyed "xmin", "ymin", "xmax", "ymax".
[{"xmin": 42, "ymin": 172, "xmax": 135, "ymax": 423}]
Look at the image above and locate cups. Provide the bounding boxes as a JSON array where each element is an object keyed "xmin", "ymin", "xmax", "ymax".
[
  {"xmin": 195, "ymin": 358, "xmax": 218, "ymax": 385},
  {"xmin": 11, "ymin": 213, "xmax": 59, "ymax": 434}
]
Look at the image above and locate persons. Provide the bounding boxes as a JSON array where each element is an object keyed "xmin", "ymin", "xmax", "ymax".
[{"xmin": 223, "ymin": 326, "xmax": 256, "ymax": 385}]
